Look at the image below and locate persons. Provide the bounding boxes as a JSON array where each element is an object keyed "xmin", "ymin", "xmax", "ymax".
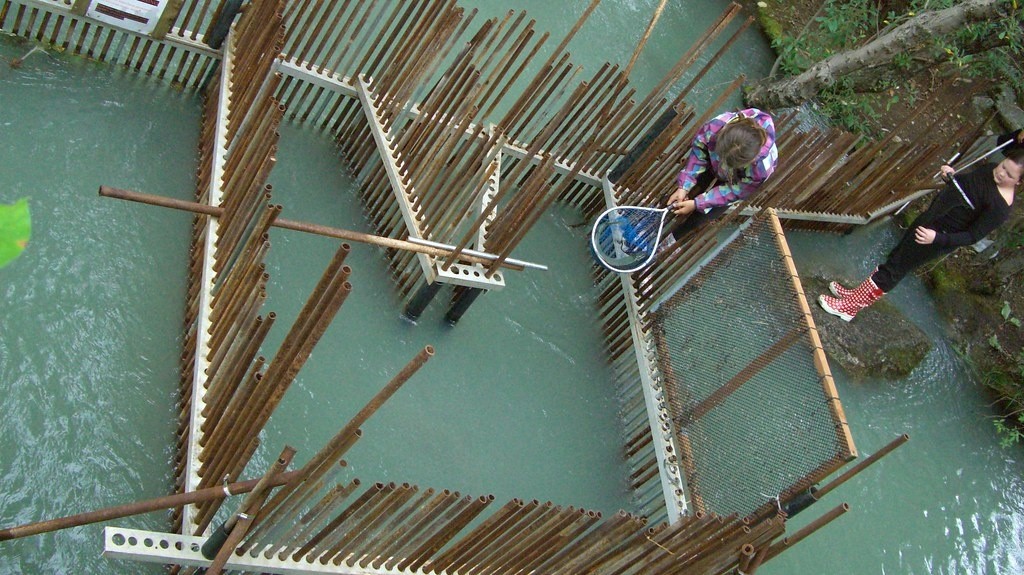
[
  {"xmin": 816, "ymin": 151, "xmax": 1024, "ymax": 321},
  {"xmin": 633, "ymin": 108, "xmax": 778, "ymax": 265}
]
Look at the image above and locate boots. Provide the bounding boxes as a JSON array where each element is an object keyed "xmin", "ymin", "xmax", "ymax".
[
  {"xmin": 637, "ymin": 233, "xmax": 682, "ymax": 298},
  {"xmin": 817, "ymin": 274, "xmax": 888, "ymax": 322},
  {"xmin": 829, "ymin": 265, "xmax": 880, "ymax": 299},
  {"xmin": 632, "ymin": 225, "xmax": 655, "ymax": 253}
]
